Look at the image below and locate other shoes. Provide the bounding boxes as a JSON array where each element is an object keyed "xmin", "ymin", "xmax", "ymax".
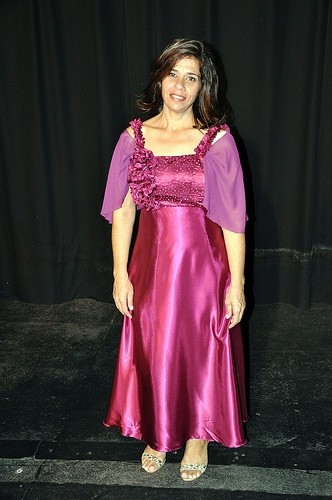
[
  {"xmin": 141, "ymin": 452, "xmax": 166, "ymax": 473},
  {"xmin": 180, "ymin": 464, "xmax": 207, "ymax": 482}
]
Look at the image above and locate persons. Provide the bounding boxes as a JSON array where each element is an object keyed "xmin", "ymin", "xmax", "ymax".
[{"xmin": 112, "ymin": 39, "xmax": 246, "ymax": 481}]
